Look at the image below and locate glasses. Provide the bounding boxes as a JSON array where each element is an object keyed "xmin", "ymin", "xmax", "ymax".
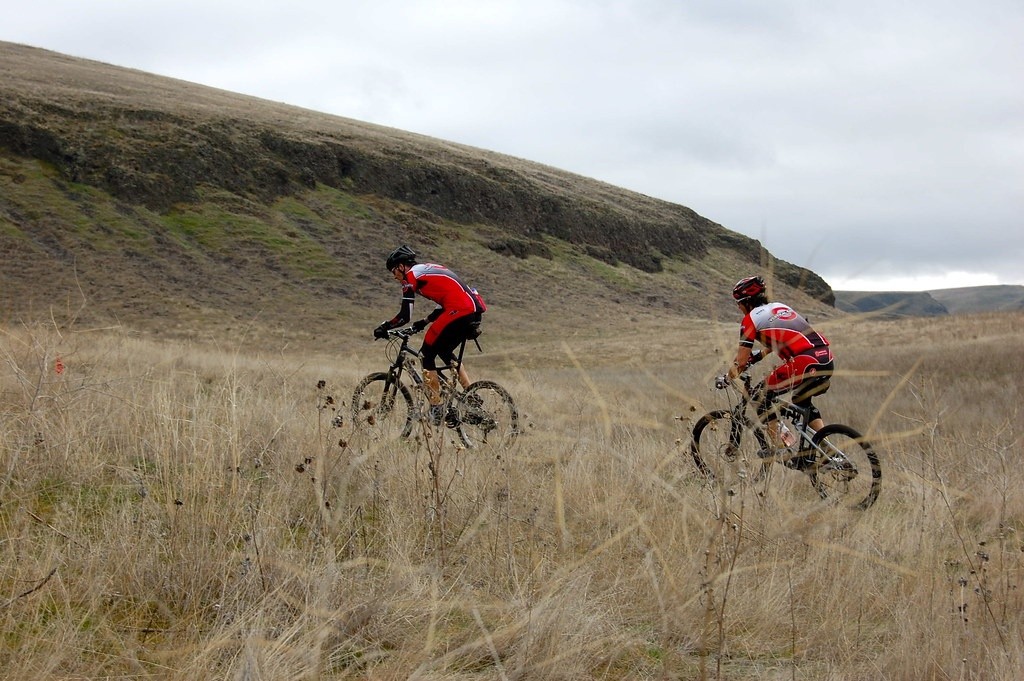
[
  {"xmin": 735, "ymin": 296, "xmax": 752, "ymax": 307},
  {"xmin": 390, "ymin": 266, "xmax": 399, "ymax": 275}
]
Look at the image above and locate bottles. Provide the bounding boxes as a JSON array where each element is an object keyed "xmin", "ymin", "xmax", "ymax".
[{"xmin": 778, "ymin": 421, "xmax": 796, "ymax": 447}]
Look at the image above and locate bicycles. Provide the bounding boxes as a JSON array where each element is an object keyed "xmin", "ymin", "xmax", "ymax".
[
  {"xmin": 350, "ymin": 324, "xmax": 520, "ymax": 455},
  {"xmin": 689, "ymin": 363, "xmax": 883, "ymax": 514}
]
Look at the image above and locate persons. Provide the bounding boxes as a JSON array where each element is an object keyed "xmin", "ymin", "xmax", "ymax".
[
  {"xmin": 716, "ymin": 276, "xmax": 834, "ymax": 468},
  {"xmin": 374, "ymin": 245, "xmax": 484, "ymax": 423}
]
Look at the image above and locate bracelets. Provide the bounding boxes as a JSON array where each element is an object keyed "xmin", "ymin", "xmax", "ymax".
[{"xmin": 764, "ymin": 351, "xmax": 767, "ymax": 356}]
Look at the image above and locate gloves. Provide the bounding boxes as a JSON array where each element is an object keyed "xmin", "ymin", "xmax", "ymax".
[
  {"xmin": 374, "ymin": 320, "xmax": 392, "ymax": 341},
  {"xmin": 412, "ymin": 319, "xmax": 427, "ymax": 333}
]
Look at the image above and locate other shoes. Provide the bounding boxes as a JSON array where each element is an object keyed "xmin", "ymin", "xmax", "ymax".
[
  {"xmin": 424, "ymin": 405, "xmax": 444, "ymax": 424},
  {"xmin": 457, "ymin": 395, "xmax": 483, "ymax": 408},
  {"xmin": 757, "ymin": 449, "xmax": 787, "ymax": 464}
]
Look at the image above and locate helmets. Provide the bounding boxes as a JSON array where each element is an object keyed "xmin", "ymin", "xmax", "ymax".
[
  {"xmin": 386, "ymin": 245, "xmax": 417, "ymax": 272},
  {"xmin": 733, "ymin": 276, "xmax": 768, "ymax": 301}
]
